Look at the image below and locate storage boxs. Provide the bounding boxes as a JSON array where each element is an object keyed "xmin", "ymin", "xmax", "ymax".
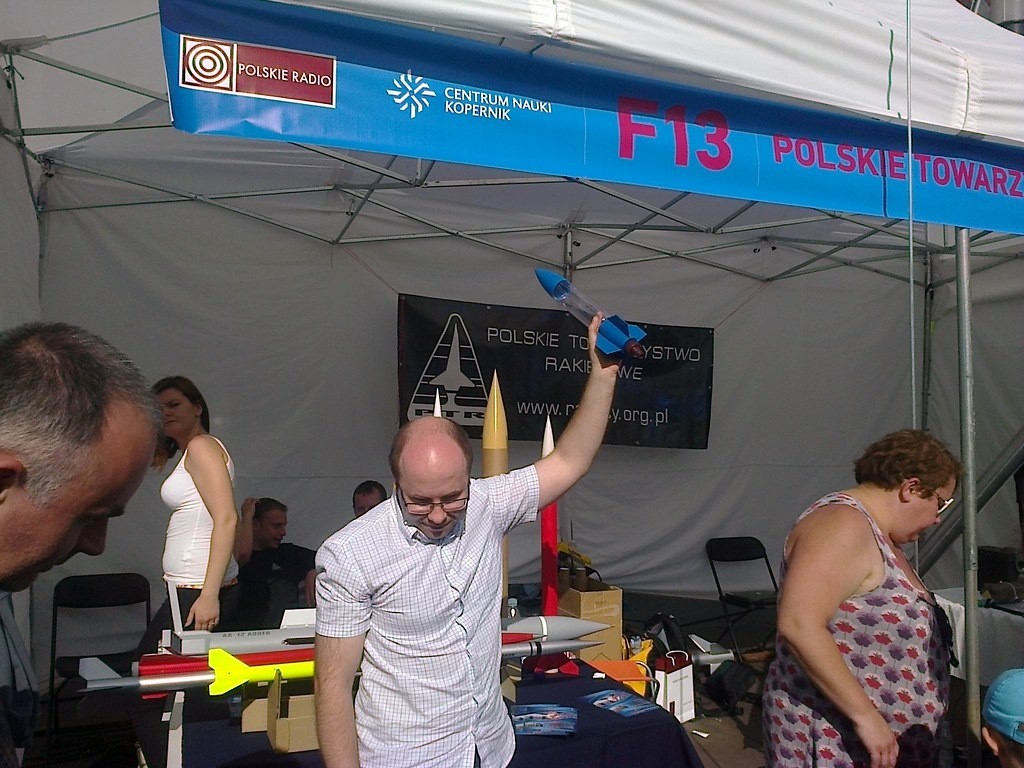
[
  {"xmin": 236, "ymin": 677, "xmax": 326, "ymax": 756},
  {"xmin": 558, "ymin": 567, "xmax": 624, "ymax": 660}
]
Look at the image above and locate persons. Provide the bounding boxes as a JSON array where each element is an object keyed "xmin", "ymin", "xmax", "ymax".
[
  {"xmin": 315, "ymin": 311, "xmax": 622, "ymax": 768},
  {"xmin": 236, "ymin": 497, "xmax": 318, "ymax": 631},
  {"xmin": 0, "ymin": 319, "xmax": 162, "ymax": 594},
  {"xmin": 981, "ymin": 667, "xmax": 1024, "ymax": 768},
  {"xmin": 352, "ymin": 479, "xmax": 386, "ymax": 518},
  {"xmin": 123, "ymin": 376, "xmax": 241, "ymax": 768},
  {"xmin": 760, "ymin": 427, "xmax": 963, "ymax": 768}
]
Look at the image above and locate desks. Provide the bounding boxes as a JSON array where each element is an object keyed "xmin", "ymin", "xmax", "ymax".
[
  {"xmin": 155, "ymin": 659, "xmax": 704, "ymax": 768},
  {"xmin": 929, "ymin": 587, "xmax": 1024, "ymax": 687}
]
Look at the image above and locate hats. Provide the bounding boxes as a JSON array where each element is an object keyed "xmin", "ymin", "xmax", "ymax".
[{"xmin": 981, "ymin": 668, "xmax": 1024, "ymax": 746}]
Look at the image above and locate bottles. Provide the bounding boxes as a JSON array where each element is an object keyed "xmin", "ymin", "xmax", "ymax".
[{"xmin": 507, "ymin": 598, "xmax": 522, "ymax": 618}]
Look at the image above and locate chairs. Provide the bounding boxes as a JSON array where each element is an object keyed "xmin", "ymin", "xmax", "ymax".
[
  {"xmin": 31, "ymin": 574, "xmax": 150, "ymax": 763},
  {"xmin": 706, "ymin": 536, "xmax": 778, "ymax": 663},
  {"xmin": 583, "ymin": 652, "xmax": 660, "ymax": 706}
]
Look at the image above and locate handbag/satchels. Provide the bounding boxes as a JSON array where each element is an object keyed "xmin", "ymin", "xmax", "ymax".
[
  {"xmin": 654, "ymin": 650, "xmax": 695, "ymax": 724},
  {"xmin": 706, "ymin": 659, "xmax": 756, "ymax": 708}
]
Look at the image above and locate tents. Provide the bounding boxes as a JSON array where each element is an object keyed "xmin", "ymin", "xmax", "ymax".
[{"xmin": 0, "ymin": 0, "xmax": 1024, "ymax": 768}]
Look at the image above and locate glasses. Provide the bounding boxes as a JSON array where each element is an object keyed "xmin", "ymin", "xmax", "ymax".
[
  {"xmin": 900, "ymin": 473, "xmax": 955, "ymax": 515},
  {"xmin": 396, "ymin": 479, "xmax": 472, "ymax": 515}
]
